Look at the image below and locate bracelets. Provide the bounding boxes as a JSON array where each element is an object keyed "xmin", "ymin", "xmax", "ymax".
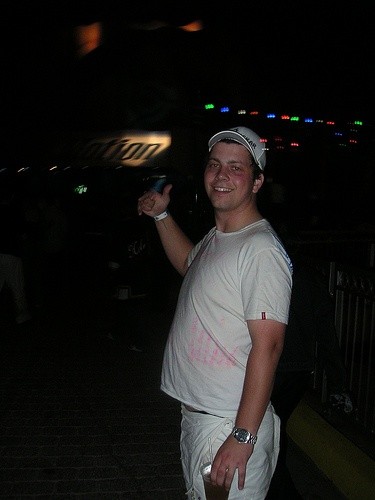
[{"xmin": 153, "ymin": 211, "xmax": 169, "ymax": 222}]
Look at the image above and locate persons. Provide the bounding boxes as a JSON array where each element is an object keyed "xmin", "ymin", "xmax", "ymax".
[
  {"xmin": 0, "ymin": 189, "xmax": 66, "ymax": 325},
  {"xmin": 137, "ymin": 127, "xmax": 294, "ymax": 499}
]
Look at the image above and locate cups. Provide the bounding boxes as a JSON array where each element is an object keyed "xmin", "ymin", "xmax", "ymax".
[{"xmin": 200, "ymin": 462, "xmax": 232, "ymax": 500}]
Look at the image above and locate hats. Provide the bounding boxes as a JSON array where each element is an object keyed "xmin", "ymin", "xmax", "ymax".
[{"xmin": 208, "ymin": 126, "xmax": 266, "ymax": 170}]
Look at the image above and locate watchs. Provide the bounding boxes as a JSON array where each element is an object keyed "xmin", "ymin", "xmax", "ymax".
[{"xmin": 230, "ymin": 427, "xmax": 257, "ymax": 445}]
